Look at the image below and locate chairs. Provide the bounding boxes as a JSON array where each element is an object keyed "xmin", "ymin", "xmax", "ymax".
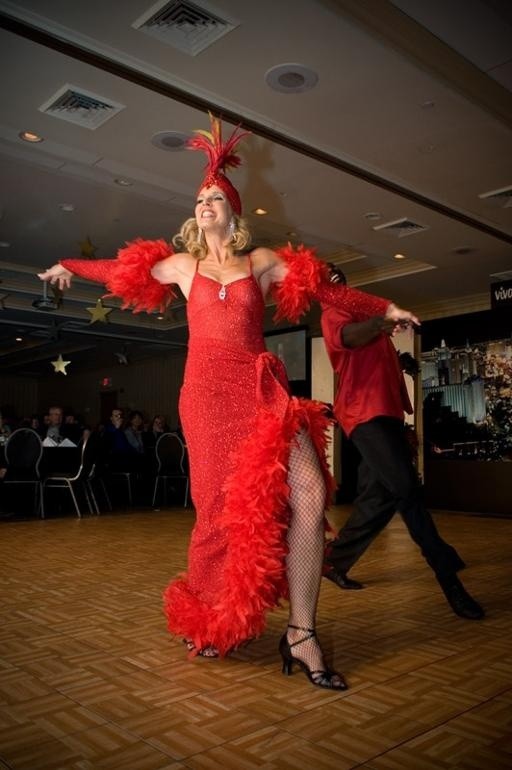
[{"xmin": 2, "ymin": 429, "xmax": 189, "ymax": 517}]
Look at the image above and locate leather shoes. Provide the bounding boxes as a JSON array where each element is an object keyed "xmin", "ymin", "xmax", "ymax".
[
  {"xmin": 438, "ymin": 572, "xmax": 486, "ymax": 619},
  {"xmin": 324, "ymin": 566, "xmax": 363, "ymax": 591}
]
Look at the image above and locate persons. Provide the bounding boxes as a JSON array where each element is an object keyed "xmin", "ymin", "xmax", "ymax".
[
  {"xmin": 0, "ymin": 405, "xmax": 181, "ymax": 518},
  {"xmin": 319, "ymin": 263, "xmax": 485, "ymax": 620},
  {"xmin": 39, "ymin": 174, "xmax": 423, "ymax": 690}
]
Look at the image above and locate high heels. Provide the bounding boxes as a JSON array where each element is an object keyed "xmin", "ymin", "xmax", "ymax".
[
  {"xmin": 278, "ymin": 625, "xmax": 347, "ymax": 691},
  {"xmin": 183, "ymin": 632, "xmax": 221, "ymax": 658}
]
